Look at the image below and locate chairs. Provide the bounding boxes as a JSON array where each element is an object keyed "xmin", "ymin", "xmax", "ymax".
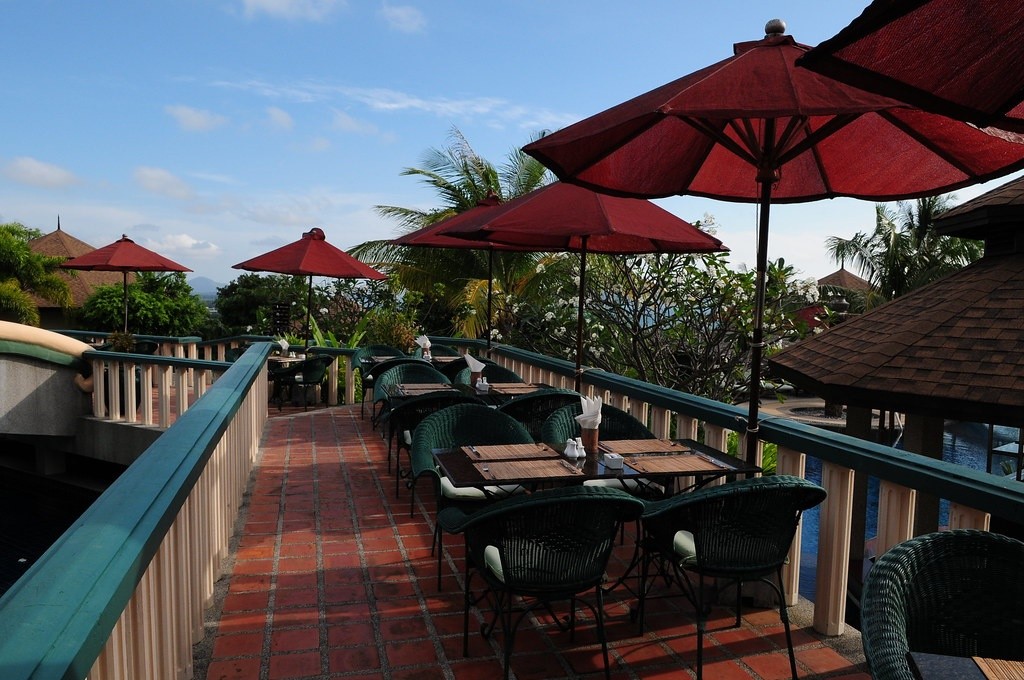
[
  {"xmin": 128, "ymin": 339, "xmax": 157, "ymax": 356},
  {"xmin": 411, "ymin": 404, "xmax": 537, "ymax": 594},
  {"xmin": 860, "ymin": 529, "xmax": 1024, "ymax": 680},
  {"xmin": 540, "ymin": 400, "xmax": 677, "ymax": 549},
  {"xmin": 350, "ymin": 341, "xmax": 587, "ymax": 519},
  {"xmin": 222, "ymin": 346, "xmax": 333, "ymax": 412},
  {"xmin": 435, "ymin": 488, "xmax": 647, "ymax": 679},
  {"xmin": 628, "ymin": 474, "xmax": 826, "ymax": 680}
]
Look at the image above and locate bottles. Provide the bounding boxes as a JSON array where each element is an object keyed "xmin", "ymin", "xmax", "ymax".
[
  {"xmin": 577, "ymin": 442, "xmax": 586, "ymax": 457},
  {"xmin": 423, "ymin": 352, "xmax": 427, "ymax": 358},
  {"xmin": 567, "ymin": 441, "xmax": 578, "ymax": 457},
  {"xmin": 428, "ymin": 351, "xmax": 431, "ymax": 358},
  {"xmin": 483, "ymin": 377, "xmax": 487, "ymax": 383},
  {"xmin": 293, "ymin": 352, "xmax": 296, "ymax": 357},
  {"xmin": 289, "ymin": 352, "xmax": 292, "ymax": 357},
  {"xmin": 564, "ymin": 439, "xmax": 572, "ymax": 454},
  {"xmin": 476, "ymin": 378, "xmax": 482, "ymax": 388}
]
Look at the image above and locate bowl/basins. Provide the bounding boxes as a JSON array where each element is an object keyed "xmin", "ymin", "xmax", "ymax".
[
  {"xmin": 298, "ymin": 354, "xmax": 306, "ymax": 358},
  {"xmin": 425, "ymin": 355, "xmax": 430, "ymax": 360},
  {"xmin": 478, "ymin": 383, "xmax": 489, "ymax": 390}
]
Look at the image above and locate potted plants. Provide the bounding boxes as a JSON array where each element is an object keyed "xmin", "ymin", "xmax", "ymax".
[{"xmin": 103, "ymin": 330, "xmax": 144, "ymax": 416}]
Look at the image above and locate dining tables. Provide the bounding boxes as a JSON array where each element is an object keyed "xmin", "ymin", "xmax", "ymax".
[
  {"xmin": 430, "ymin": 440, "xmax": 642, "ymax": 633},
  {"xmin": 85, "ymin": 343, "xmax": 106, "ymax": 350},
  {"xmin": 578, "ymin": 439, "xmax": 763, "ymax": 626},
  {"xmin": 395, "ymin": 381, "xmax": 555, "ymax": 476},
  {"xmin": 267, "ymin": 355, "xmax": 305, "ymax": 403},
  {"xmin": 357, "ymin": 357, "xmax": 463, "ymax": 373}
]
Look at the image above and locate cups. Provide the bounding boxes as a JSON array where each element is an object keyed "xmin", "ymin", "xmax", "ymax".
[
  {"xmin": 282, "ymin": 349, "xmax": 288, "ymax": 356},
  {"xmin": 422, "ymin": 348, "xmax": 428, "ymax": 359},
  {"xmin": 582, "ymin": 427, "xmax": 599, "ymax": 453},
  {"xmin": 471, "ymin": 372, "xmax": 481, "ymax": 387}
]
